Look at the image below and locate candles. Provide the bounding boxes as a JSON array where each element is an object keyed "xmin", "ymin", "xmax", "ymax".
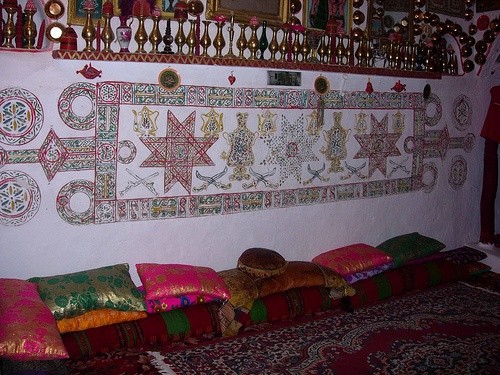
[{"xmin": 366, "ymin": 0, "xmax": 415, "ymax": 43}]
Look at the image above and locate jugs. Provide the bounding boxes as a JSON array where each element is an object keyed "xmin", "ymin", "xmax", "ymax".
[{"xmin": 116, "ymin": 15, "xmax": 135, "ymax": 53}]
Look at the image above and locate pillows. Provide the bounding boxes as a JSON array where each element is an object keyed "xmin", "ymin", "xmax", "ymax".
[
  {"xmin": 0, "ymin": 278, "xmax": 70, "ymax": 361},
  {"xmin": 136, "ymin": 285, "xmax": 219, "ymax": 314},
  {"xmin": 26, "ymin": 263, "xmax": 147, "ymax": 321},
  {"xmin": 56, "ymin": 310, "xmax": 147, "ymax": 334},
  {"xmin": 405, "ymin": 252, "xmax": 444, "ymax": 266},
  {"xmin": 135, "ymin": 263, "xmax": 231, "ymax": 301},
  {"xmin": 346, "ymin": 263, "xmax": 394, "ymax": 285},
  {"xmin": 378, "ymin": 232, "xmax": 446, "ymax": 269},
  {"xmin": 217, "ymin": 261, "xmax": 346, "ymax": 309},
  {"xmin": 311, "ymin": 244, "xmax": 391, "ymax": 277}
]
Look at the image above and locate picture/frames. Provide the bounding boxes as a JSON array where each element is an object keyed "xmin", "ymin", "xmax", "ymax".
[
  {"xmin": 155, "ymin": 0, "xmax": 187, "ymax": 20},
  {"xmin": 205, "ymin": 0, "xmax": 291, "ymax": 30},
  {"xmin": 301, "ymin": 0, "xmax": 353, "ymax": 38},
  {"xmin": 68, "ymin": 0, "xmax": 108, "ymax": 28},
  {"xmin": 425, "ymin": 0, "xmax": 465, "ymax": 18}
]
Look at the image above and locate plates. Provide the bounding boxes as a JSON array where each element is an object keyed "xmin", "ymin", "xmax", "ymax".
[
  {"xmin": 423, "ymin": 84, "xmax": 432, "ymax": 99},
  {"xmin": 158, "ymin": 68, "xmax": 181, "ymax": 92},
  {"xmin": 314, "ymin": 75, "xmax": 331, "ymax": 95}
]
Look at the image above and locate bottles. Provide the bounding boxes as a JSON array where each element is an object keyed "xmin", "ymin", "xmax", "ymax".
[
  {"xmin": 82, "ymin": 7, "xmax": 96, "ymax": 51},
  {"xmin": 175, "ymin": 18, "xmax": 186, "ymax": 56},
  {"xmin": 60, "ymin": 24, "xmax": 77, "ymax": 50},
  {"xmin": 24, "ymin": 8, "xmax": 36, "ymax": 49},
  {"xmin": 259, "ymin": 20, "xmax": 268, "ymax": 59},
  {"xmin": 2, "ymin": 8, "xmax": 17, "ymax": 47},
  {"xmin": 187, "ymin": 19, "xmax": 198, "ymax": 57},
  {"xmin": 268, "ymin": 26, "xmax": 458, "ymax": 76},
  {"xmin": 213, "ymin": 22, "xmax": 225, "ymax": 58},
  {"xmin": 162, "ymin": 19, "xmax": 175, "ymax": 54},
  {"xmin": 249, "ymin": 23, "xmax": 260, "ymax": 59},
  {"xmin": 235, "ymin": 23, "xmax": 249, "ymax": 59},
  {"xmin": 101, "ymin": 12, "xmax": 115, "ymax": 52},
  {"xmin": 149, "ymin": 15, "xmax": 163, "ymax": 54},
  {"xmin": 135, "ymin": 15, "xmax": 147, "ymax": 54},
  {"xmin": 200, "ymin": 21, "xmax": 212, "ymax": 57}
]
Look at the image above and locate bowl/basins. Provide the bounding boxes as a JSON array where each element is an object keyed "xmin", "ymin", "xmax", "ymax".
[
  {"xmin": 353, "ymin": 0, "xmax": 365, "ymax": 42},
  {"xmin": 416, "ymin": 0, "xmax": 500, "ymax": 73},
  {"xmin": 371, "ymin": 1, "xmax": 409, "ymax": 33},
  {"xmin": 188, "ymin": 0, "xmax": 204, "ymax": 16},
  {"xmin": 290, "ymin": 0, "xmax": 301, "ymax": 26}
]
